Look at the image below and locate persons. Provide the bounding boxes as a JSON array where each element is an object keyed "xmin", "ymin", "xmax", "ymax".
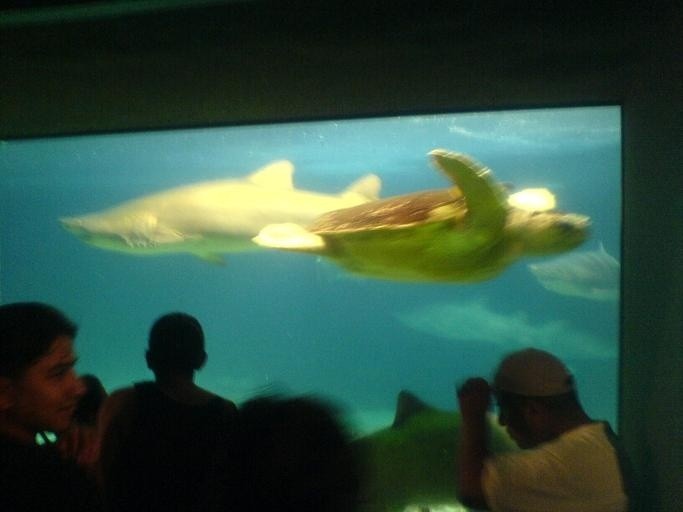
[{"xmin": 0, "ymin": 302, "xmax": 636, "ymax": 512}]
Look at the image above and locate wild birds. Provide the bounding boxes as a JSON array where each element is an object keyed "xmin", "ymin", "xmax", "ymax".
[{"xmin": 525, "ymin": 238, "xmax": 621, "ymax": 301}]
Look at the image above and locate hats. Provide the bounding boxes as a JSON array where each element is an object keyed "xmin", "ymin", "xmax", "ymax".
[{"xmin": 486, "ymin": 349, "xmax": 573, "ymax": 397}]
